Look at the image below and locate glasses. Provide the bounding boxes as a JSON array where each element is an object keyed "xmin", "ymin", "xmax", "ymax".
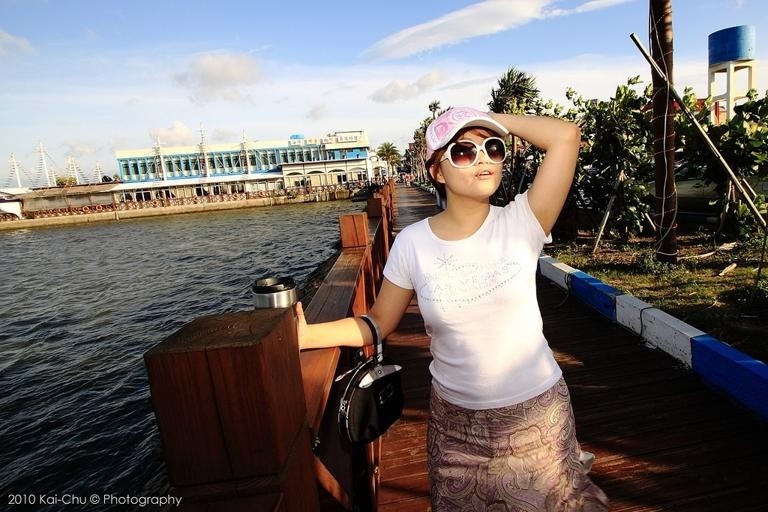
[{"xmin": 438, "ymin": 136, "xmax": 507, "ymax": 168}]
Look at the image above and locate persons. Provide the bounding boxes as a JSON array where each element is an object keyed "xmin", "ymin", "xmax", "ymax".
[{"xmin": 291, "ymin": 106, "xmax": 614, "ymax": 511}]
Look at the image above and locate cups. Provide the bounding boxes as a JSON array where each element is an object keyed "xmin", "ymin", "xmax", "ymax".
[{"xmin": 251, "ymin": 276, "xmax": 300, "ymax": 322}]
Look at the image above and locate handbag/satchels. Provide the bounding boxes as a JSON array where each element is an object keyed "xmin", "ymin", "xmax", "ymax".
[{"xmin": 334, "ymin": 314, "xmax": 404, "ymax": 451}]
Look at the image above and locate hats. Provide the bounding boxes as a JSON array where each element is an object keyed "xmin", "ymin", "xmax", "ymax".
[{"xmin": 424, "ymin": 106, "xmax": 509, "ymax": 162}]
